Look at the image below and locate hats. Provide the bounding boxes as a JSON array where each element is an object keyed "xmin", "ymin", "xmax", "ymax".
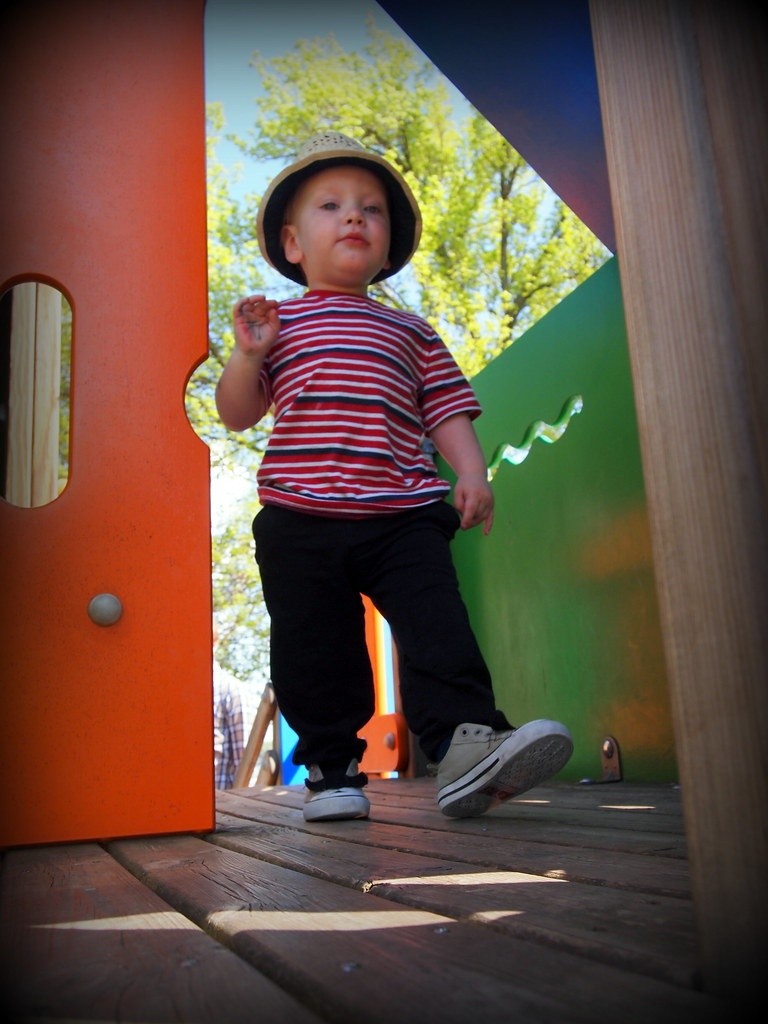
[{"xmin": 255, "ymin": 132, "xmax": 423, "ymax": 288}]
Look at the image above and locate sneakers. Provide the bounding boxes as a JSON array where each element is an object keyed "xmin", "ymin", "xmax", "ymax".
[
  {"xmin": 303, "ymin": 758, "xmax": 371, "ymax": 822},
  {"xmin": 436, "ymin": 718, "xmax": 572, "ymax": 818}
]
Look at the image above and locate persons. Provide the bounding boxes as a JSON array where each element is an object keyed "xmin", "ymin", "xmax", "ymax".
[
  {"xmin": 214, "ymin": 131, "xmax": 575, "ymax": 822},
  {"xmin": 213, "ymin": 615, "xmax": 243, "ymax": 791}
]
